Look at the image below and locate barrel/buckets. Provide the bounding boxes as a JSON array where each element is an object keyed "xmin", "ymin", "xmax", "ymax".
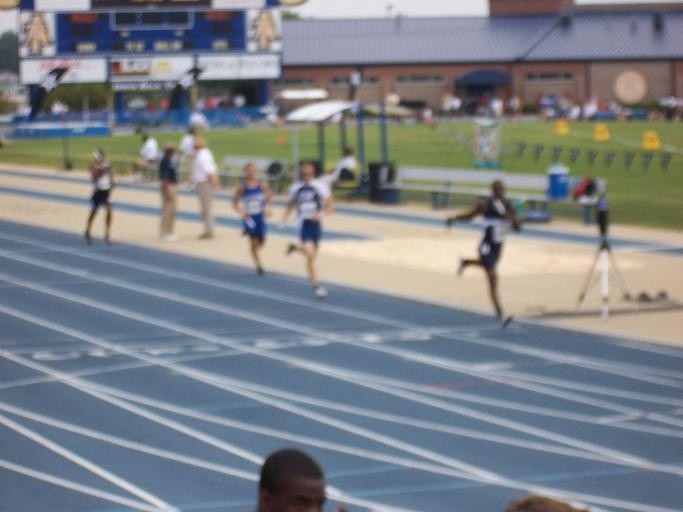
[{"xmin": 547, "ymin": 167, "xmax": 571, "ymax": 199}]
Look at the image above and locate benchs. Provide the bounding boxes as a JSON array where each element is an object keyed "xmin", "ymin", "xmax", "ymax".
[
  {"xmin": 378, "ymin": 164, "xmax": 607, "ymax": 226},
  {"xmin": 213, "ymin": 156, "xmax": 288, "ymax": 195}
]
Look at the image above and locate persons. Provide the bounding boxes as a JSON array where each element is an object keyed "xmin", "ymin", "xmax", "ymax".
[
  {"xmin": 128, "ymin": 105, "xmax": 217, "ymax": 245},
  {"xmin": 231, "ymin": 163, "xmax": 273, "ymax": 278},
  {"xmin": 411, "ymin": 91, "xmax": 682, "ymax": 129},
  {"xmin": 281, "ymin": 159, "xmax": 335, "ymax": 299},
  {"xmin": 446, "ymin": 179, "xmax": 521, "ymax": 328},
  {"xmin": 251, "ymin": 448, "xmax": 349, "ymax": 512},
  {"xmin": 330, "ymin": 145, "xmax": 361, "ymax": 184},
  {"xmin": 84, "ymin": 148, "xmax": 115, "ymax": 245}
]
